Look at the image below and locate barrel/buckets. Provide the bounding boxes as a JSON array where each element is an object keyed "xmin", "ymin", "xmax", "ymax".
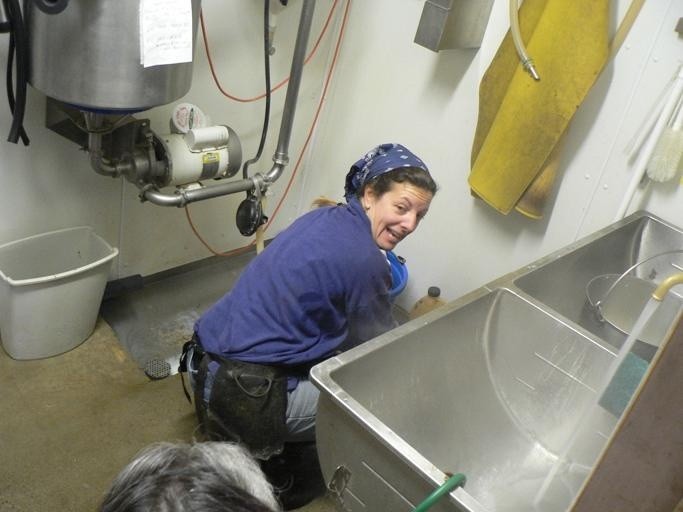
[
  {"xmin": 383, "ymin": 251, "xmax": 406, "ymax": 295},
  {"xmin": 579, "ymin": 249, "xmax": 683, "ymax": 362},
  {"xmin": 410, "ymin": 287, "xmax": 445, "ymax": 320}
]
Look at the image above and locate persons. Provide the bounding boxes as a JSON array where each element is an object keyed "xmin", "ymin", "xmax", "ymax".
[
  {"xmin": 95, "ymin": 436, "xmax": 283, "ymax": 512},
  {"xmin": 177, "ymin": 142, "xmax": 439, "ymax": 502}
]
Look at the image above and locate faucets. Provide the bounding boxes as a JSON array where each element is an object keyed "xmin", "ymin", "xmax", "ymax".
[{"xmin": 651, "ymin": 273, "xmax": 683, "ymax": 302}]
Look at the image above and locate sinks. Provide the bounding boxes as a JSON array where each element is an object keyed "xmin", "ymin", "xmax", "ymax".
[
  {"xmin": 311, "ymin": 288, "xmax": 649, "ymax": 512},
  {"xmin": 513, "ymin": 210, "xmax": 683, "ymax": 368}
]
